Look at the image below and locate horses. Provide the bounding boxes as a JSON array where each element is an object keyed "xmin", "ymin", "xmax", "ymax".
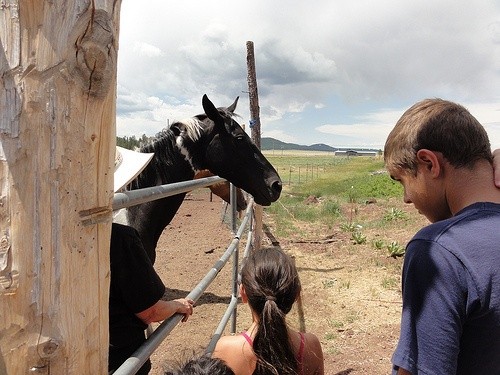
[{"xmin": 112, "ymin": 94, "xmax": 283, "ymax": 267}]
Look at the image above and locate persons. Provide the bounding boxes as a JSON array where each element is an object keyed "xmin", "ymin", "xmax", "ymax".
[
  {"xmin": 212, "ymin": 248, "xmax": 324, "ymax": 375},
  {"xmin": 108, "ymin": 146, "xmax": 193, "ymax": 375},
  {"xmin": 383, "ymin": 98, "xmax": 500, "ymax": 375}
]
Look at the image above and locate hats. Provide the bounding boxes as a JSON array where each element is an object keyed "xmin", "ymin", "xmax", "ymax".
[{"xmin": 114, "ymin": 146, "xmax": 157, "ymax": 192}]
